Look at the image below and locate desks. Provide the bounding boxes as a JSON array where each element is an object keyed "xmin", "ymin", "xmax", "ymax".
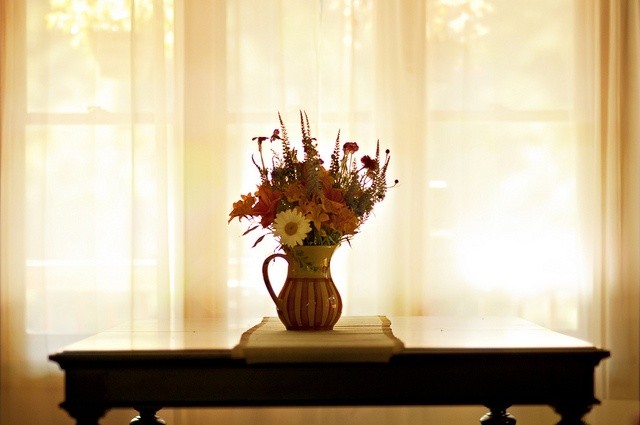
[{"xmin": 46, "ymin": 310, "xmax": 612, "ymax": 425}]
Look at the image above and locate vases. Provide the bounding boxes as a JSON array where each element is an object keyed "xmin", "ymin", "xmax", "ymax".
[{"xmin": 259, "ymin": 245, "xmax": 342, "ymax": 331}]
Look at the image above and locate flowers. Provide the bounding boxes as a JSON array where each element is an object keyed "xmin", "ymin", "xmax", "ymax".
[{"xmin": 225, "ymin": 108, "xmax": 399, "ymax": 245}]
